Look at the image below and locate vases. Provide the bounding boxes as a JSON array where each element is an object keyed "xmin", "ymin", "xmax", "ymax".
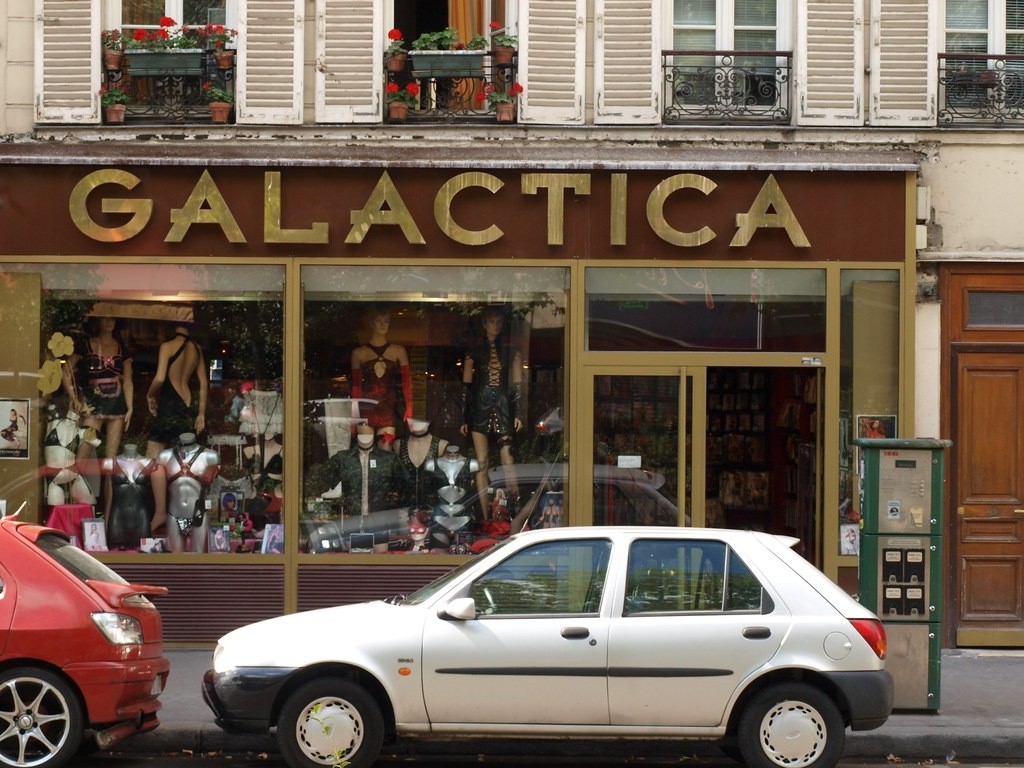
[
  {"xmin": 387, "ymin": 53, "xmax": 407, "ymax": 72},
  {"xmin": 388, "ymin": 102, "xmax": 410, "ymax": 120},
  {"xmin": 256, "ymin": 434, "xmax": 265, "ymax": 491},
  {"xmin": 744, "ymin": 72, "xmax": 776, "ymax": 98},
  {"xmin": 212, "ymin": 50, "xmax": 234, "ymax": 69},
  {"xmin": 407, "ymin": 49, "xmax": 488, "ymax": 78},
  {"xmin": 208, "ymin": 101, "xmax": 233, "ymax": 124},
  {"xmin": 493, "ymin": 45, "xmax": 514, "ymax": 66},
  {"xmin": 496, "ymin": 102, "xmax": 515, "ymax": 122},
  {"xmin": 978, "ymin": 71, "xmax": 996, "ymax": 88},
  {"xmin": 103, "ymin": 103, "xmax": 127, "ymax": 123},
  {"xmin": 103, "ymin": 48, "xmax": 123, "ymax": 71},
  {"xmin": 123, "ymin": 48, "xmax": 205, "ymax": 76},
  {"xmin": 953, "ymin": 69, "xmax": 974, "ymax": 89}
]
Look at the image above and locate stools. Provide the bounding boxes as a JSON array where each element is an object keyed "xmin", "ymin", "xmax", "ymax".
[{"xmin": 206, "ymin": 435, "xmax": 247, "ymax": 471}]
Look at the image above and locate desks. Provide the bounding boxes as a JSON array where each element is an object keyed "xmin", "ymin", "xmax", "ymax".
[{"xmin": 41, "ymin": 503, "xmax": 95, "ymax": 550}]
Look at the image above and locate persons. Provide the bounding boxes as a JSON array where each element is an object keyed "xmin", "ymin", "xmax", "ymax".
[
  {"xmin": 539, "ymin": 498, "xmax": 560, "ymax": 528},
  {"xmin": 489, "ymin": 488, "xmax": 511, "ymax": 537},
  {"xmin": 322, "ymin": 433, "xmax": 411, "ymax": 515},
  {"xmin": 266, "ymin": 524, "xmax": 284, "ymax": 554},
  {"xmin": 155, "ymin": 432, "xmax": 221, "ymax": 553},
  {"xmin": 351, "ymin": 304, "xmax": 414, "ymax": 452},
  {"xmin": 45, "ymin": 317, "xmax": 134, "ymax": 531},
  {"xmin": 459, "ymin": 306, "xmax": 524, "ymax": 521},
  {"xmin": 864, "ymin": 419, "xmax": 886, "ymax": 438},
  {"xmin": 150, "ymin": 539, "xmax": 166, "ymax": 553},
  {"xmin": 147, "ymin": 322, "xmax": 208, "ymax": 531},
  {"xmin": 243, "ymin": 433, "xmax": 284, "ymax": 482},
  {"xmin": 0, "ymin": 408, "xmax": 26, "ymax": 450},
  {"xmin": 393, "ymin": 417, "xmax": 450, "ymax": 479},
  {"xmin": 408, "ymin": 505, "xmax": 429, "ymax": 552},
  {"xmin": 423, "ymin": 445, "xmax": 480, "ymax": 487},
  {"xmin": 215, "ymin": 529, "xmax": 226, "ymax": 550},
  {"xmin": 221, "ymin": 493, "xmax": 238, "ymax": 519},
  {"xmin": 101, "ymin": 444, "xmax": 158, "ymax": 547},
  {"xmin": 85, "ymin": 524, "xmax": 101, "ymax": 549}
]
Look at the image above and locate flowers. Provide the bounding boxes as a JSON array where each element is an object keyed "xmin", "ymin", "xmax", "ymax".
[
  {"xmin": 127, "ymin": 15, "xmax": 209, "ymax": 49},
  {"xmin": 383, "ymin": 82, "xmax": 419, "ymax": 109},
  {"xmin": 383, "ymin": 28, "xmax": 408, "ymax": 59},
  {"xmin": 489, "ymin": 20, "xmax": 518, "ymax": 48},
  {"xmin": 477, "ymin": 81, "xmax": 523, "ymax": 114},
  {"xmin": 101, "ymin": 28, "xmax": 128, "ymax": 51},
  {"xmin": 411, "ymin": 27, "xmax": 490, "ymax": 50},
  {"xmin": 205, "ymin": 22, "xmax": 239, "ymax": 57},
  {"xmin": 203, "ymin": 81, "xmax": 234, "ymax": 103},
  {"xmin": 98, "ymin": 82, "xmax": 129, "ymax": 109},
  {"xmin": 240, "ymin": 377, "xmax": 283, "ymax": 433}
]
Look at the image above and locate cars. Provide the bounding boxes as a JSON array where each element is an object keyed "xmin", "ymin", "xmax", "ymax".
[
  {"xmin": 0, "ymin": 501, "xmax": 171, "ymax": 768},
  {"xmin": 310, "ymin": 464, "xmax": 692, "ymax": 553},
  {"xmin": 203, "ymin": 525, "xmax": 894, "ymax": 768},
  {"xmin": 303, "ymin": 398, "xmax": 380, "ymax": 424}
]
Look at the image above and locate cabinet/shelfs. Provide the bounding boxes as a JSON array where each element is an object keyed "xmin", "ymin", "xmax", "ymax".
[
  {"xmin": 778, "ymin": 386, "xmax": 804, "ymax": 530},
  {"xmin": 804, "ymin": 374, "xmax": 825, "ymax": 530},
  {"xmin": 594, "ymin": 375, "xmax": 693, "ymax": 526},
  {"xmin": 706, "ymin": 367, "xmax": 773, "ymax": 534}
]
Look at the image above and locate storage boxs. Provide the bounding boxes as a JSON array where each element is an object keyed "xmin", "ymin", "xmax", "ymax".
[
  {"xmin": 140, "ymin": 538, "xmax": 172, "ymax": 554},
  {"xmin": 260, "ymin": 524, "xmax": 284, "ymax": 555},
  {"xmin": 207, "ymin": 526, "xmax": 230, "ymax": 552}
]
